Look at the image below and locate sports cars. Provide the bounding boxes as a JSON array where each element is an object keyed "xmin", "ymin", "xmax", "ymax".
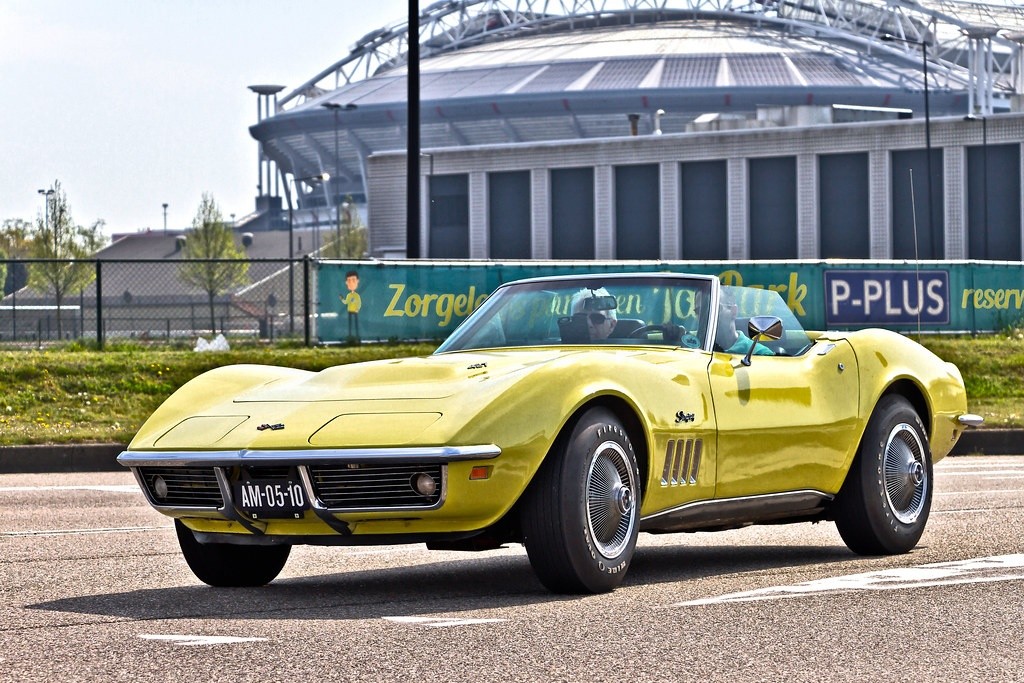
[{"xmin": 114, "ymin": 269, "xmax": 985, "ymax": 597}]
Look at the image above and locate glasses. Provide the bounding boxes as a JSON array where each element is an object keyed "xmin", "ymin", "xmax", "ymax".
[
  {"xmin": 693, "ymin": 302, "xmax": 731, "ymax": 315},
  {"xmin": 573, "ymin": 313, "xmax": 613, "ymax": 324}
]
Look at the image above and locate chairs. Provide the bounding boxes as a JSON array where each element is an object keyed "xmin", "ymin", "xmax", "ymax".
[
  {"xmin": 609, "ymin": 319, "xmax": 647, "ymax": 338},
  {"xmin": 736, "ymin": 318, "xmax": 789, "ymax": 354}
]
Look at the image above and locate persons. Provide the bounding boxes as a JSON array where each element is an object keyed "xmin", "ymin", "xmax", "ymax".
[
  {"xmin": 663, "ymin": 281, "xmax": 777, "ymax": 356},
  {"xmin": 569, "ymin": 288, "xmax": 617, "ymax": 338}
]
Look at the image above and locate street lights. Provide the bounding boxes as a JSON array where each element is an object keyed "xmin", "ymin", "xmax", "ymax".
[
  {"xmin": 37, "ymin": 188, "xmax": 55, "ymax": 247},
  {"xmin": 163, "ymin": 203, "xmax": 169, "ymax": 231}
]
